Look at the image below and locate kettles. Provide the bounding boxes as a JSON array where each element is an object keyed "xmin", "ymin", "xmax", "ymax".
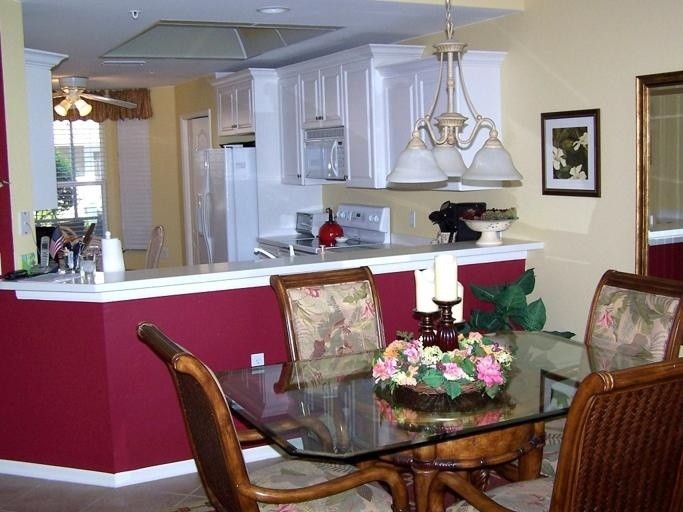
[{"xmin": 318, "ymin": 207, "xmax": 343, "ymax": 243}]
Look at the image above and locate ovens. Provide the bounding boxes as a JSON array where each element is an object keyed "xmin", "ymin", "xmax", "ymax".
[{"xmin": 304, "ymin": 132, "xmax": 348, "ymax": 181}]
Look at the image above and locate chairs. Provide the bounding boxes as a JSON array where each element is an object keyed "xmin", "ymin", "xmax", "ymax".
[
  {"xmin": 426, "ymin": 355, "xmax": 683, "ymax": 511},
  {"xmin": 145, "ymin": 224, "xmax": 166, "ymax": 269},
  {"xmin": 136, "ymin": 319, "xmax": 410, "ymax": 511},
  {"xmin": 584, "ymin": 270, "xmax": 683, "ymax": 359},
  {"xmin": 270, "ymin": 268, "xmax": 387, "ymax": 362}
]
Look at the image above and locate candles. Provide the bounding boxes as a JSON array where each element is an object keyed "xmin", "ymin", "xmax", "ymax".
[
  {"xmin": 435, "ymin": 254, "xmax": 458, "ymax": 302},
  {"xmin": 415, "ymin": 269, "xmax": 438, "ymax": 313},
  {"xmin": 451, "ymin": 283, "xmax": 464, "ymax": 323}
]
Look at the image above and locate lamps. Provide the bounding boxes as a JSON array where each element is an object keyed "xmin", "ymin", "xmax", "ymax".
[
  {"xmin": 49, "ymin": 75, "xmax": 139, "ymax": 118},
  {"xmin": 386, "ymin": 0, "xmax": 525, "ymax": 184}
]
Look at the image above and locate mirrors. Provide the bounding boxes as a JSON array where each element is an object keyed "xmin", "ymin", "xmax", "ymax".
[{"xmin": 634, "ymin": 69, "xmax": 683, "ymax": 277}]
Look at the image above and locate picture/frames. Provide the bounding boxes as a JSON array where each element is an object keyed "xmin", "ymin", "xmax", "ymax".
[{"xmin": 541, "ymin": 107, "xmax": 603, "ymax": 198}]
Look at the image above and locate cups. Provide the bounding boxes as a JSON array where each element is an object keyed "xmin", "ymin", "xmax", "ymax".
[{"xmin": 79, "ymin": 251, "xmax": 96, "ymax": 273}]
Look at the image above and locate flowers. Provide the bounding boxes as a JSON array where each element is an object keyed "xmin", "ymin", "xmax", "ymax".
[{"xmin": 374, "ymin": 334, "xmax": 512, "ymax": 403}]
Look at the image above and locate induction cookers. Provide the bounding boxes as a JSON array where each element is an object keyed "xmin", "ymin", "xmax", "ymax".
[{"xmin": 280, "ymin": 204, "xmax": 390, "ymax": 254}]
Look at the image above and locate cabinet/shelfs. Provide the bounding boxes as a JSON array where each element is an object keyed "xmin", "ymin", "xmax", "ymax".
[
  {"xmin": 389, "ymin": 66, "xmax": 503, "ymax": 192},
  {"xmin": 25, "ymin": 48, "xmax": 70, "ymax": 210},
  {"xmin": 280, "ymin": 68, "xmax": 388, "ymax": 190},
  {"xmin": 217, "ymin": 81, "xmax": 256, "ymax": 136}
]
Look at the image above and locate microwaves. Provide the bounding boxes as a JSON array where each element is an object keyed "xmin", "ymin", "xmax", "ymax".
[{"xmin": 295, "ymin": 212, "xmax": 317, "ymax": 237}]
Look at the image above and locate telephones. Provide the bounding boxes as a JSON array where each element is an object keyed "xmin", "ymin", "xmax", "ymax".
[{"xmin": 30, "ymin": 235, "xmax": 58, "ymax": 276}]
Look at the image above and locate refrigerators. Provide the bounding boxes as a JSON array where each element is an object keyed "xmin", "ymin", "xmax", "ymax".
[{"xmin": 191, "ymin": 147, "xmax": 258, "ymax": 265}]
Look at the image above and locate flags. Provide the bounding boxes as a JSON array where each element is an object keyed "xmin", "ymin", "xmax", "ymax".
[{"xmin": 47, "ymin": 224, "xmax": 66, "ymax": 259}]
